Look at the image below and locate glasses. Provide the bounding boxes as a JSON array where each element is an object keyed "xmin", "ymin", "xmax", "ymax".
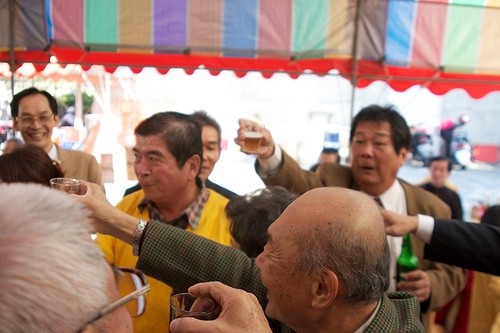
[
  {"xmin": 74, "ymin": 267, "xmax": 151, "ymax": 333},
  {"xmin": 17, "ymin": 113, "xmax": 53, "ymax": 126}
]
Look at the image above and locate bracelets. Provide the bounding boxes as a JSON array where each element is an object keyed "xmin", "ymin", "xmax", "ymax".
[{"xmin": 132, "ymin": 219, "xmax": 147, "ymax": 256}]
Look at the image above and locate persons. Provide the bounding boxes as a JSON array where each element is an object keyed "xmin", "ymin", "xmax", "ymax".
[
  {"xmin": 381, "ymin": 209, "xmax": 500, "ymax": 276},
  {"xmin": 0, "ymin": 181, "xmax": 272, "ymax": 333},
  {"xmin": 420, "ymin": 156, "xmax": 463, "ymax": 220},
  {"xmin": 223, "ymin": 185, "xmax": 299, "ymax": 259},
  {"xmin": 438, "ymin": 115, "xmax": 469, "ymax": 157},
  {"xmin": 0, "ymin": 144, "xmax": 65, "ymax": 189},
  {"xmin": 309, "ymin": 147, "xmax": 341, "ymax": 173},
  {"xmin": 124, "ymin": 111, "xmax": 236, "ymax": 199},
  {"xmin": 93, "ymin": 110, "xmax": 232, "ymax": 333},
  {"xmin": 2, "ymin": 138, "xmax": 22, "ymax": 155},
  {"xmin": 60, "ymin": 179, "xmax": 425, "ymax": 333},
  {"xmin": 234, "ymin": 104, "xmax": 468, "ymax": 333},
  {"xmin": 10, "ymin": 87, "xmax": 105, "ymax": 195}
]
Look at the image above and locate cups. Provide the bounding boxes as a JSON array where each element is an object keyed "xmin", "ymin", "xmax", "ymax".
[
  {"xmin": 239, "ymin": 124, "xmax": 264, "ymax": 154},
  {"xmin": 169, "ymin": 293, "xmax": 217, "ymax": 321},
  {"xmin": 50, "ymin": 177, "xmax": 82, "ymax": 195}
]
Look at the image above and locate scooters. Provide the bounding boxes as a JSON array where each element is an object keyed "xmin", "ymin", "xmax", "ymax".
[
  {"xmin": 410, "ymin": 121, "xmax": 435, "ymax": 167},
  {"xmin": 439, "ymin": 114, "xmax": 472, "ymax": 171}
]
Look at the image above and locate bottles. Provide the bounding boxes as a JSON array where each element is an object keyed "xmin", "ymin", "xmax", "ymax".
[{"xmin": 396, "ymin": 234, "xmax": 420, "ymax": 291}]
